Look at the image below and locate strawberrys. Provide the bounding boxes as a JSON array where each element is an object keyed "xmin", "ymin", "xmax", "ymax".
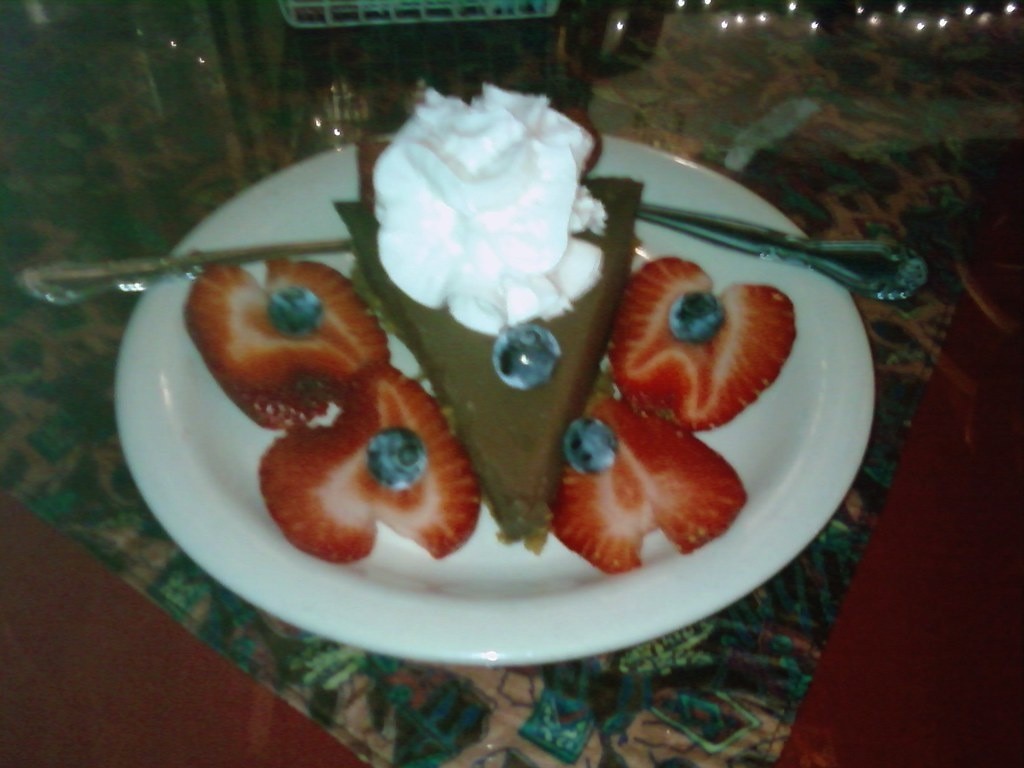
[
  {"xmin": 186, "ymin": 251, "xmax": 395, "ymax": 430},
  {"xmin": 259, "ymin": 365, "xmax": 481, "ymax": 558},
  {"xmin": 553, "ymin": 386, "xmax": 750, "ymax": 573},
  {"xmin": 604, "ymin": 259, "xmax": 798, "ymax": 429}
]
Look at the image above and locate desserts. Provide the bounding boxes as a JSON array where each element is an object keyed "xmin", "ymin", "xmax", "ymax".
[{"xmin": 329, "ymin": 91, "xmax": 651, "ymax": 559}]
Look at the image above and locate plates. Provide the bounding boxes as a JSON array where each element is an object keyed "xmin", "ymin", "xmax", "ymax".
[{"xmin": 114, "ymin": 137, "xmax": 876, "ymax": 670}]
